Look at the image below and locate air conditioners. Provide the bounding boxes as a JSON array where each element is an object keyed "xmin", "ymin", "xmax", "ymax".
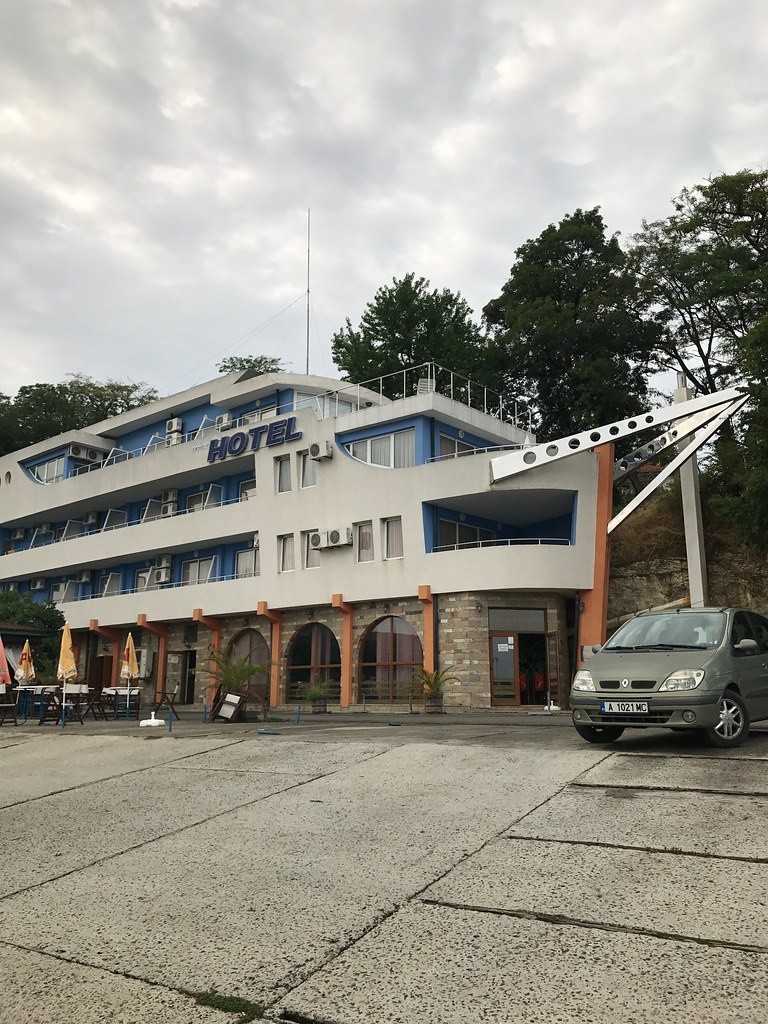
[
  {"xmin": 4, "ymin": 583, "xmax": 18, "ymax": 592},
  {"xmin": 67, "ymin": 413, "xmax": 351, "ymax": 584},
  {"xmin": 11, "ymin": 527, "xmax": 25, "ymax": 541},
  {"xmin": 30, "ymin": 577, "xmax": 45, "ymax": 589},
  {"xmin": 36, "ymin": 522, "xmax": 50, "ymax": 535}
]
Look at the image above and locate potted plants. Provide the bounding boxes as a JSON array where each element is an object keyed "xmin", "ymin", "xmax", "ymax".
[
  {"xmin": 409, "ymin": 665, "xmax": 461, "ymax": 714},
  {"xmin": 300, "ymin": 673, "xmax": 340, "ymax": 713}
]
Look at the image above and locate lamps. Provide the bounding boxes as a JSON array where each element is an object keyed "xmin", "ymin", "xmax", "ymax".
[
  {"xmin": 476, "ymin": 602, "xmax": 482, "ymax": 612},
  {"xmin": 383, "ymin": 604, "xmax": 389, "ymax": 613},
  {"xmin": 308, "ymin": 610, "xmax": 313, "ymax": 620},
  {"xmin": 242, "ymin": 617, "xmax": 248, "ymax": 626},
  {"xmin": 578, "ymin": 600, "xmax": 584, "ymax": 612},
  {"xmin": 184, "ymin": 639, "xmax": 190, "ymax": 648}
]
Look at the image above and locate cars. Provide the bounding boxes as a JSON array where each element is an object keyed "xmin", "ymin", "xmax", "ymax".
[{"xmin": 568, "ymin": 605, "xmax": 768, "ymax": 748}]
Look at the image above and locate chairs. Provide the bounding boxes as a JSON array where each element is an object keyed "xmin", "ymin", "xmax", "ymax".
[
  {"xmin": 743, "ymin": 624, "xmax": 762, "ymax": 648},
  {"xmin": 659, "ymin": 630, "xmax": 679, "ymax": 645},
  {"xmin": 155, "ymin": 682, "xmax": 181, "ymax": 720},
  {"xmin": 0, "ymin": 682, "xmax": 131, "ymax": 727}
]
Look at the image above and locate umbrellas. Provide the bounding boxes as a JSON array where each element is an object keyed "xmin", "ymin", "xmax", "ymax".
[
  {"xmin": 121, "ymin": 632, "xmax": 139, "ymax": 721},
  {"xmin": 57, "ymin": 624, "xmax": 77, "ymax": 729},
  {"xmin": 14, "ymin": 639, "xmax": 35, "ymax": 705},
  {"xmin": 0, "ymin": 635, "xmax": 12, "ymax": 727}
]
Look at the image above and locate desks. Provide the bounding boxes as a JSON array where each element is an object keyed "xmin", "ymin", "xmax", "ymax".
[{"xmin": 12, "ymin": 685, "xmax": 143, "ymax": 724}]
